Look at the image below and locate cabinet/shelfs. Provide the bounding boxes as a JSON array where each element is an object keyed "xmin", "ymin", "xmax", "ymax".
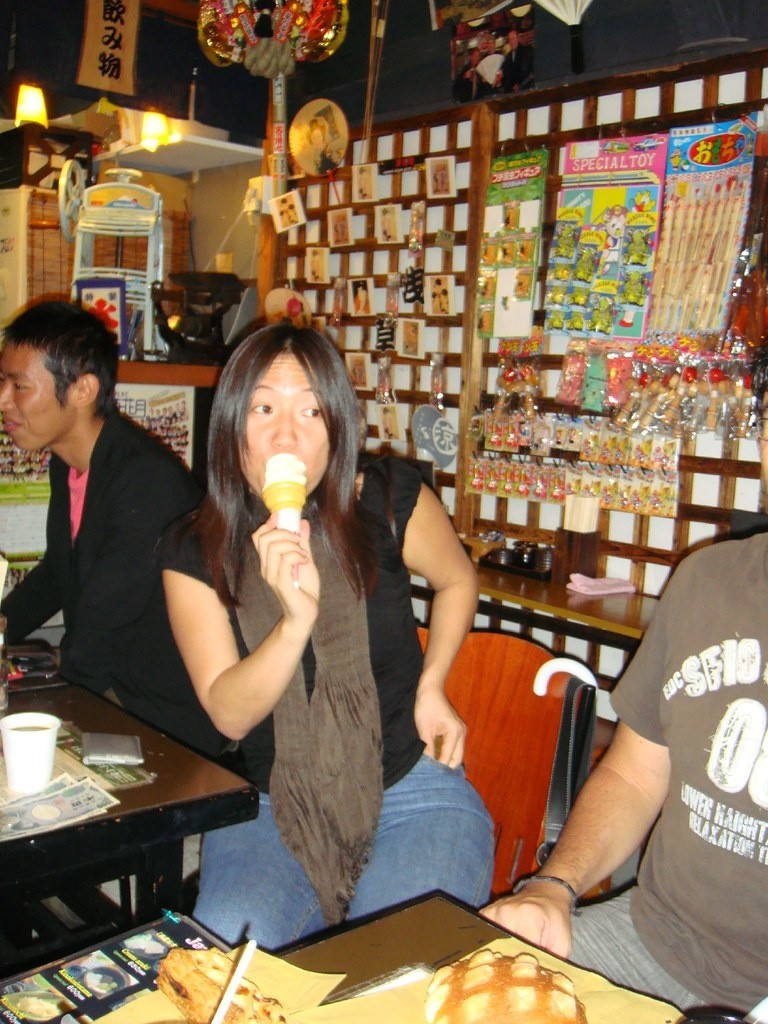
[{"xmin": 83, "ymin": 137, "xmax": 267, "ymax": 391}]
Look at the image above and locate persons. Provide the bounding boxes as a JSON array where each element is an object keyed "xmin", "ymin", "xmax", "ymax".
[
  {"xmin": 479, "ymin": 380, "xmax": 767, "ymax": 1020},
  {"xmin": 0, "ymin": 300, "xmax": 232, "ymax": 760},
  {"xmin": 154, "ymin": 323, "xmax": 496, "ymax": 954}
]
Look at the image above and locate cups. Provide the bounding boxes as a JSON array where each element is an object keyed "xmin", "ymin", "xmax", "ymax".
[
  {"xmin": 534, "ymin": 544, "xmax": 553, "ymax": 572},
  {"xmin": 513, "ymin": 540, "xmax": 538, "ymax": 569},
  {"xmin": 493, "ymin": 545, "xmax": 513, "ymax": 565},
  {"xmin": 0, "ymin": 712, "xmax": 61, "ymax": 795}
]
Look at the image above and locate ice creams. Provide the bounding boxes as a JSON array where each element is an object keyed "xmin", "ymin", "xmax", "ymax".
[{"xmin": 262, "ymin": 455, "xmax": 307, "ymax": 589}]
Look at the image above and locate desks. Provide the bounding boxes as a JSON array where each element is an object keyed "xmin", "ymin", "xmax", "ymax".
[
  {"xmin": 0, "ymin": 680, "xmax": 258, "ymax": 982},
  {"xmin": 462, "ymin": 550, "xmax": 657, "ymax": 638},
  {"xmin": 272, "ymin": 888, "xmax": 680, "ymax": 1024}
]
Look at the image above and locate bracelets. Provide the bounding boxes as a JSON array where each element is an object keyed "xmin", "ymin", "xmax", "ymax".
[{"xmin": 512, "ymin": 876, "xmax": 576, "ymax": 911}]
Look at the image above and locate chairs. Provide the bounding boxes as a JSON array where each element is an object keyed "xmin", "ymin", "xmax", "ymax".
[{"xmin": 414, "ymin": 622, "xmax": 594, "ymax": 905}]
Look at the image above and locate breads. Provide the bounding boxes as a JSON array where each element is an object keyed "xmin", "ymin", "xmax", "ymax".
[
  {"xmin": 427, "ymin": 950, "xmax": 588, "ymax": 1024},
  {"xmin": 157, "ymin": 948, "xmax": 289, "ymax": 1024}
]
